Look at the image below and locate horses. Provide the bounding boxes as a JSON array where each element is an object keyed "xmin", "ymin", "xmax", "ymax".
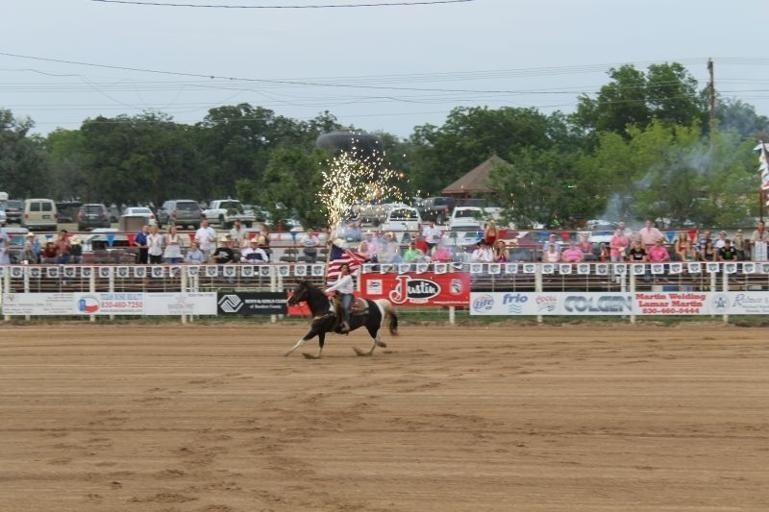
[{"xmin": 283, "ymin": 279, "xmax": 399, "ymax": 358}]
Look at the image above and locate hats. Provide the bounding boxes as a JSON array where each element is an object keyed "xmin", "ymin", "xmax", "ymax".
[
  {"xmin": 248, "ymin": 238, "xmax": 259, "ymax": 243},
  {"xmin": 25, "ymin": 232, "xmax": 35, "ymax": 238}
]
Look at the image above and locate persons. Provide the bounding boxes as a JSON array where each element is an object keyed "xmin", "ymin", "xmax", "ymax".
[
  {"xmin": 323, "ymin": 262, "xmax": 354, "ymax": 332},
  {"xmin": 543, "ymin": 215, "xmax": 769, "ymax": 282},
  {"xmin": 300, "ymin": 220, "xmax": 510, "ymax": 263},
  {"xmin": 0, "ymin": 214, "xmax": 274, "ymax": 282}
]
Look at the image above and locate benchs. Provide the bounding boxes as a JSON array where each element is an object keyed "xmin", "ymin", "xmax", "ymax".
[{"xmin": 470, "ymin": 268, "xmax": 769, "ymax": 292}]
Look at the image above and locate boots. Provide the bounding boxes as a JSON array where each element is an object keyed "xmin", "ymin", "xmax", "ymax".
[{"xmin": 340, "ymin": 321, "xmax": 351, "ymax": 332}]
